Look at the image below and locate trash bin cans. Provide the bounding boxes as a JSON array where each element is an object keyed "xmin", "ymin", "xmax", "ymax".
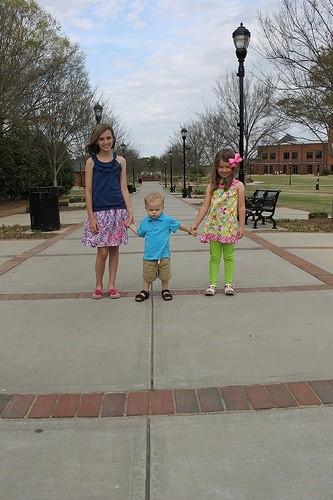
[
  {"xmin": 28, "ymin": 186, "xmax": 62, "ymax": 232},
  {"xmin": 138, "ymin": 178, "xmax": 143, "ymax": 183},
  {"xmin": 127, "ymin": 184, "xmax": 133, "ymax": 194}
]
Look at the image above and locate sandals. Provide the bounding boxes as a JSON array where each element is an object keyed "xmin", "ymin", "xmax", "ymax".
[
  {"xmin": 204, "ymin": 285, "xmax": 217, "ymax": 295},
  {"xmin": 223, "ymin": 283, "xmax": 235, "ymax": 294},
  {"xmin": 135, "ymin": 290, "xmax": 149, "ymax": 301},
  {"xmin": 161, "ymin": 289, "xmax": 172, "ymax": 301}
]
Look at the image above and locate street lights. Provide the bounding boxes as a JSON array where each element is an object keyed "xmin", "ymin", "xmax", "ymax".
[
  {"xmin": 169, "ymin": 150, "xmax": 173, "ymax": 192},
  {"xmin": 289, "ymin": 163, "xmax": 292, "ymax": 185},
  {"xmin": 164, "ymin": 160, "xmax": 167, "ymax": 187},
  {"xmin": 181, "ymin": 128, "xmax": 188, "ymax": 198},
  {"xmin": 232, "ymin": 22, "xmax": 251, "ymax": 185}
]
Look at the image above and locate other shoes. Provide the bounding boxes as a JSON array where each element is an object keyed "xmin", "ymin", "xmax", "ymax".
[
  {"xmin": 107, "ymin": 288, "xmax": 119, "ymax": 299},
  {"xmin": 91, "ymin": 288, "xmax": 103, "ymax": 299}
]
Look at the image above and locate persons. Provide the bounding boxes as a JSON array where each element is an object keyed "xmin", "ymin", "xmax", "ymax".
[
  {"xmin": 189, "ymin": 149, "xmax": 245, "ymax": 295},
  {"xmin": 80, "ymin": 125, "xmax": 135, "ymax": 299},
  {"xmin": 125, "ymin": 192, "xmax": 198, "ymax": 302}
]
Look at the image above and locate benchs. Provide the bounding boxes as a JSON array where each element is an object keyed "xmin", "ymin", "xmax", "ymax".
[{"xmin": 238, "ymin": 188, "xmax": 281, "ymax": 229}]
